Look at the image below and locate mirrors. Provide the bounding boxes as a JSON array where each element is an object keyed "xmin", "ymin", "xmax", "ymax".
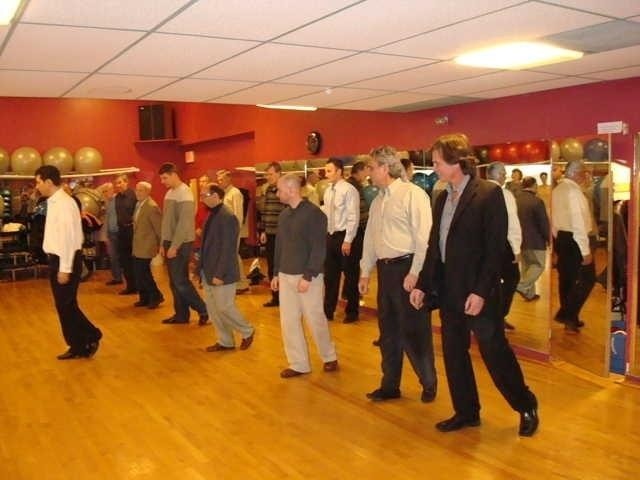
[
  {"xmin": 549, "ymin": 135, "xmax": 610, "ymax": 376},
  {"xmin": 307, "ymin": 158, "xmax": 334, "ymax": 205},
  {"xmin": 412, "ymin": 166, "xmax": 434, "ymax": 191},
  {"xmin": 499, "ymin": 161, "xmax": 550, "ymax": 364},
  {"xmin": 255, "ymin": 160, "xmax": 306, "ymax": 287},
  {"xmin": 341, "ymin": 155, "xmax": 378, "ymax": 313}
]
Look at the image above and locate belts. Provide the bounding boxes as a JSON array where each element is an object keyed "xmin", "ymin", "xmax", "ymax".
[{"xmin": 379, "ymin": 255, "xmax": 412, "ymax": 264}]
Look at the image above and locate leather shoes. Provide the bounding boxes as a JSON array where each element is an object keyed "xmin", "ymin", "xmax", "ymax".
[
  {"xmin": 366, "ymin": 388, "xmax": 400, "ymax": 399},
  {"xmin": 515, "ymin": 288, "xmax": 540, "ymax": 303},
  {"xmin": 105, "ymin": 280, "xmax": 164, "ymax": 310},
  {"xmin": 325, "ymin": 359, "xmax": 336, "ymax": 374},
  {"xmin": 438, "ymin": 411, "xmax": 481, "ymax": 432},
  {"xmin": 555, "ymin": 309, "xmax": 583, "ymax": 332},
  {"xmin": 421, "ymin": 377, "xmax": 438, "ymax": 403},
  {"xmin": 264, "ymin": 298, "xmax": 279, "ymax": 307},
  {"xmin": 519, "ymin": 394, "xmax": 538, "ymax": 437},
  {"xmin": 57, "ymin": 328, "xmax": 102, "ymax": 357},
  {"xmin": 344, "ymin": 311, "xmax": 357, "ymax": 324},
  {"xmin": 504, "ymin": 320, "xmax": 515, "ymax": 332},
  {"xmin": 204, "ymin": 341, "xmax": 237, "ymax": 352},
  {"xmin": 164, "ymin": 311, "xmax": 208, "ymax": 326},
  {"xmin": 281, "ymin": 367, "xmax": 312, "ymax": 378},
  {"xmin": 240, "ymin": 328, "xmax": 255, "ymax": 351}
]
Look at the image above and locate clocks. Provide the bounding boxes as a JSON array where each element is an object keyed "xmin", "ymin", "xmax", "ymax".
[{"xmin": 304, "ymin": 132, "xmax": 320, "ymax": 154}]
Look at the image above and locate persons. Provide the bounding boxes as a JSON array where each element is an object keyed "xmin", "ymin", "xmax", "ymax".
[
  {"xmin": 131, "ymin": 182, "xmax": 165, "ymax": 308},
  {"xmin": 194, "ymin": 158, "xmax": 434, "ymax": 324},
  {"xmin": 103, "ymin": 182, "xmax": 128, "ymax": 284},
  {"xmin": 158, "ymin": 163, "xmax": 208, "ymax": 325},
  {"xmin": 271, "ymin": 173, "xmax": 339, "ymax": 377},
  {"xmin": 482, "ymin": 158, "xmax": 629, "ymax": 334},
  {"xmin": 357, "ymin": 146, "xmax": 438, "ymax": 403},
  {"xmin": 199, "ymin": 182, "xmax": 255, "ymax": 352},
  {"xmin": 410, "ymin": 133, "xmax": 538, "ymax": 435},
  {"xmin": 114, "ymin": 175, "xmax": 142, "ymax": 294},
  {"xmin": 36, "ymin": 166, "xmax": 102, "ymax": 357}
]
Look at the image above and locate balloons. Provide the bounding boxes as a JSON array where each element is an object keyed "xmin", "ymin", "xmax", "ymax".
[
  {"xmin": 0, "ymin": 145, "xmax": 103, "ymax": 174},
  {"xmin": 487, "ymin": 138, "xmax": 611, "ymax": 165}
]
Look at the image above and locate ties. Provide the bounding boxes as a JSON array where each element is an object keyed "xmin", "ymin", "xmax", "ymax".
[{"xmin": 328, "ymin": 184, "xmax": 335, "ymax": 235}]
